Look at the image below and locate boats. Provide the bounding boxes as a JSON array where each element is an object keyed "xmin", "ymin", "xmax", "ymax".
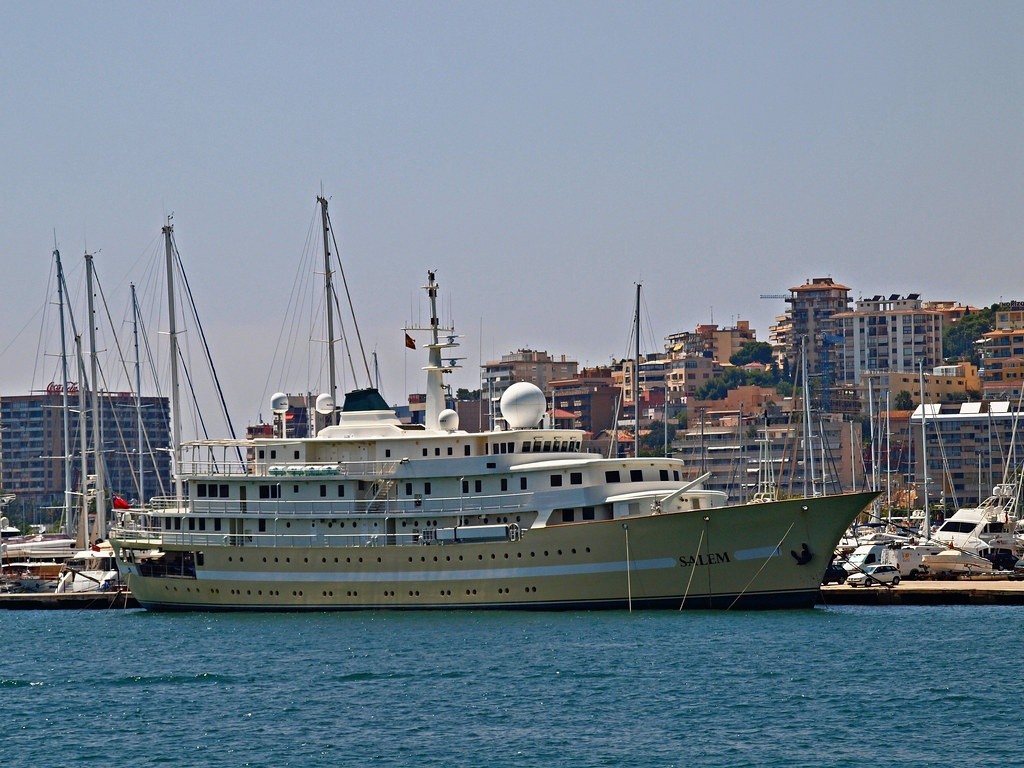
[{"xmin": 108, "ymin": 258, "xmax": 884, "ymax": 609}]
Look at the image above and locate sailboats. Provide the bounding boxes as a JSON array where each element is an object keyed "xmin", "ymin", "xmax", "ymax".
[{"xmin": 0, "ymin": 195, "xmax": 246, "ymax": 607}]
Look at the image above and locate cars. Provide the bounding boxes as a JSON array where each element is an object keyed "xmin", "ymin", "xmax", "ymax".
[{"xmin": 821, "ymin": 502, "xmax": 1024, "ymax": 588}]
